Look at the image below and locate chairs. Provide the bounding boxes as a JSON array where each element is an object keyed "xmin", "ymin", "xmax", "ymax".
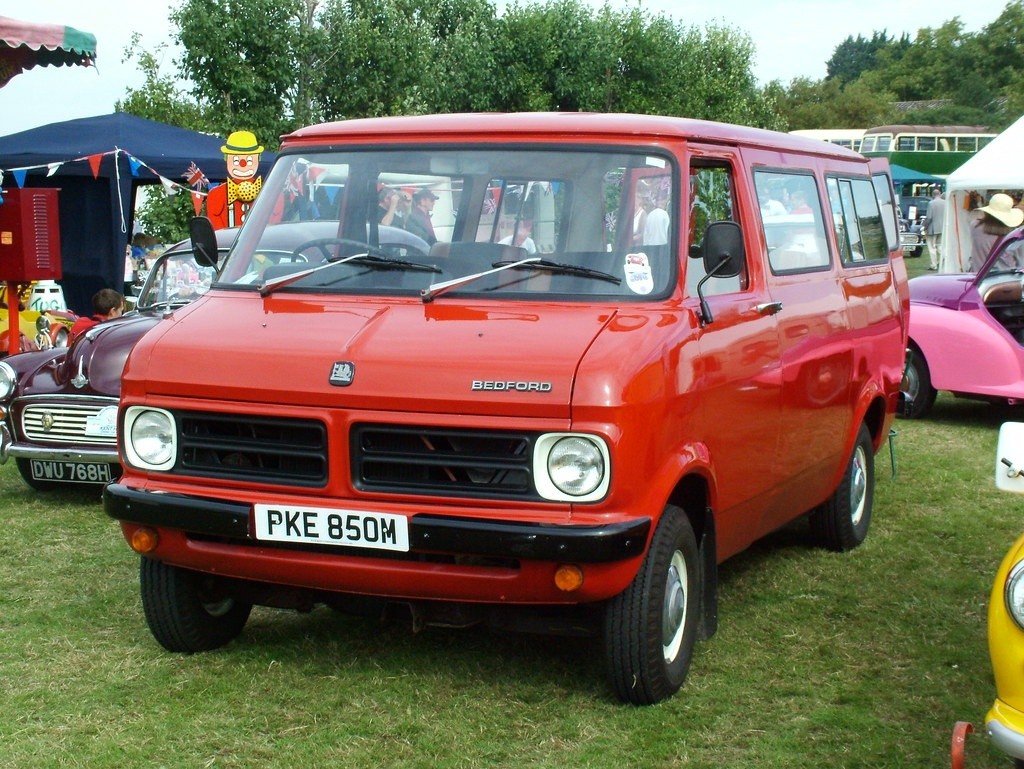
[
  {"xmin": 424, "ymin": 241, "xmax": 525, "ymax": 301},
  {"xmin": 526, "ymin": 250, "xmax": 616, "ymax": 302}
]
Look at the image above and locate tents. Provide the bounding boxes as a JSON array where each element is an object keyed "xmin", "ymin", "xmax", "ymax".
[
  {"xmin": 889, "ymin": 163, "xmax": 947, "ymax": 200},
  {"xmin": 938, "ymin": 115, "xmax": 1024, "ymax": 275},
  {"xmin": 1, "ymin": 111, "xmax": 281, "ymax": 322}
]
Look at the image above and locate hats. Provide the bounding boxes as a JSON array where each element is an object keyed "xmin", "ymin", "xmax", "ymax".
[
  {"xmin": 418, "ymin": 189, "xmax": 439, "ymax": 200},
  {"xmin": 974, "ymin": 193, "xmax": 1024, "ymax": 227}
]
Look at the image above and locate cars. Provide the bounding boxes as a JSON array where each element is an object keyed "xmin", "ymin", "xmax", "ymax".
[
  {"xmin": 0, "ymin": 218, "xmax": 442, "ymax": 494},
  {"xmin": 890, "ymin": 221, "xmax": 1024, "ymax": 416}
]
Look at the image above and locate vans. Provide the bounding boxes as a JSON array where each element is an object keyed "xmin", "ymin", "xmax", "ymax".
[{"xmin": 103, "ymin": 109, "xmax": 919, "ymax": 710}]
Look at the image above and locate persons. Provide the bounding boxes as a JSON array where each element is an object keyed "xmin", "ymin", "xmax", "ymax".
[
  {"xmin": 921, "ymin": 188, "xmax": 947, "ymax": 270},
  {"xmin": 376, "ymin": 186, "xmax": 405, "ymax": 229},
  {"xmin": 62, "ymin": 289, "xmax": 128, "ymax": 349},
  {"xmin": 644, "ymin": 196, "xmax": 671, "ymax": 246},
  {"xmin": 407, "ymin": 188, "xmax": 440, "ymax": 248},
  {"xmin": 967, "ymin": 193, "xmax": 1024, "ymax": 273},
  {"xmin": 789, "ymin": 190, "xmax": 813, "ymax": 212},
  {"xmin": 760, "ymin": 185, "xmax": 789, "ymax": 212},
  {"xmin": 632, "ymin": 192, "xmax": 648, "ymax": 245},
  {"xmin": 496, "ymin": 219, "xmax": 538, "ymax": 256}
]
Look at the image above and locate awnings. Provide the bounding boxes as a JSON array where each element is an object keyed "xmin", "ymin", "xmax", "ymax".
[{"xmin": 0, "ymin": 15, "xmax": 97, "ymax": 89}]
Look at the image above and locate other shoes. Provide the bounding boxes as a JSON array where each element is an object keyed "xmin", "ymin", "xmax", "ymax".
[{"xmin": 928, "ymin": 267, "xmax": 933, "ymax": 270}]
